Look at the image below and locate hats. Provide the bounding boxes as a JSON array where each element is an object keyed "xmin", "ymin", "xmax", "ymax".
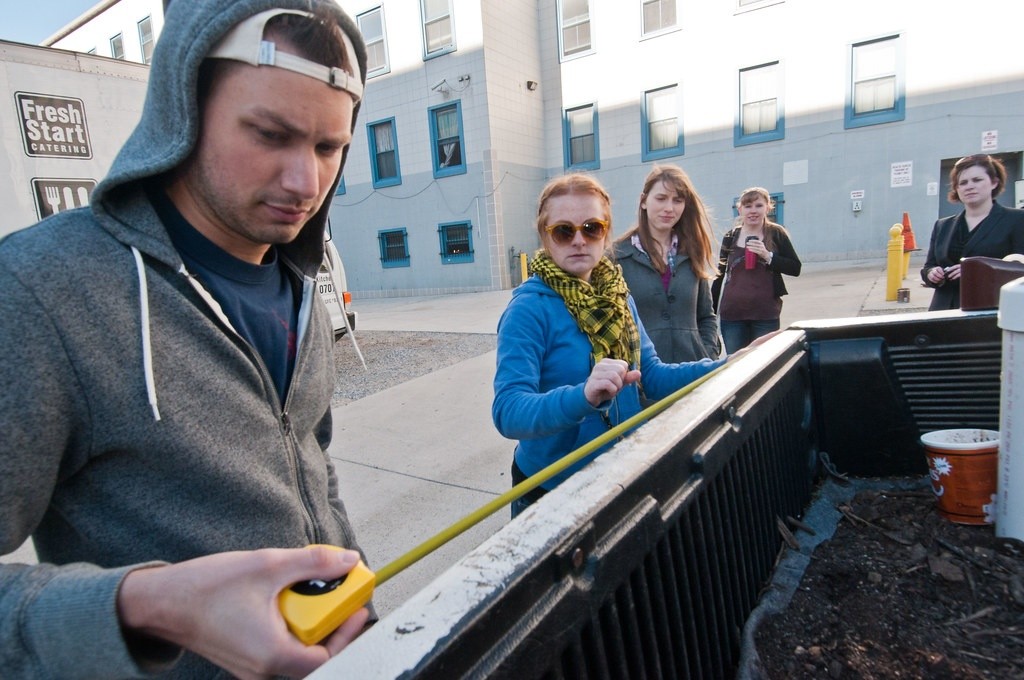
[{"xmin": 204, "ymin": 8, "xmax": 365, "ymax": 107}]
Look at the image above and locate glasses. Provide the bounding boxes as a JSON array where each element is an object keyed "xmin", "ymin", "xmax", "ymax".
[
  {"xmin": 955, "ymin": 153, "xmax": 998, "ymax": 175},
  {"xmin": 544, "ymin": 218, "xmax": 610, "ymax": 243}
]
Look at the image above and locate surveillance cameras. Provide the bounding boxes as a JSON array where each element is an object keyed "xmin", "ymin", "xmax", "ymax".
[{"xmin": 431, "ymin": 79, "xmax": 446, "ymax": 90}]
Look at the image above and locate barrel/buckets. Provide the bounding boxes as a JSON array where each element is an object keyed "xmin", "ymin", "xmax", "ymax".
[{"xmin": 919, "ymin": 429, "xmax": 1000, "ymax": 527}]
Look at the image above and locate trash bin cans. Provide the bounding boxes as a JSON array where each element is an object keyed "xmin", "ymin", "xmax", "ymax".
[{"xmin": 995, "ymin": 278, "xmax": 1024, "ymax": 555}]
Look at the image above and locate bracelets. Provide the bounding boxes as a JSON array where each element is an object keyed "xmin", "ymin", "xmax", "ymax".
[{"xmin": 766, "ymin": 252, "xmax": 773, "ymax": 265}]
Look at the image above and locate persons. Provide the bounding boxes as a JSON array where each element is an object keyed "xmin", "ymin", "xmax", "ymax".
[
  {"xmin": 0, "ymin": 1, "xmax": 379, "ymax": 680},
  {"xmin": 920, "ymin": 154, "xmax": 1024, "ymax": 311},
  {"xmin": 492, "ymin": 174, "xmax": 752, "ymax": 520},
  {"xmin": 711, "ymin": 187, "xmax": 801, "ymax": 355},
  {"xmin": 606, "ymin": 164, "xmax": 732, "ymax": 365}
]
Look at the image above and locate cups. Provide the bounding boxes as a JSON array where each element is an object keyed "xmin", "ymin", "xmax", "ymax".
[{"xmin": 744, "ymin": 236, "xmax": 759, "ymax": 270}]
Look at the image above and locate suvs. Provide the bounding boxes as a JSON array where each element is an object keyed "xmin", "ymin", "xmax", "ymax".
[{"xmin": 317, "ymin": 229, "xmax": 355, "ymax": 341}]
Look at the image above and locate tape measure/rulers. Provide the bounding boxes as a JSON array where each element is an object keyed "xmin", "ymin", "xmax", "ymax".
[{"xmin": 277, "ymin": 348, "xmax": 751, "ymax": 646}]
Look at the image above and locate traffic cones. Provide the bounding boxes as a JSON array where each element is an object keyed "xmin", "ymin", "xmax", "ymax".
[{"xmin": 902, "ymin": 213, "xmax": 922, "ymax": 252}]
